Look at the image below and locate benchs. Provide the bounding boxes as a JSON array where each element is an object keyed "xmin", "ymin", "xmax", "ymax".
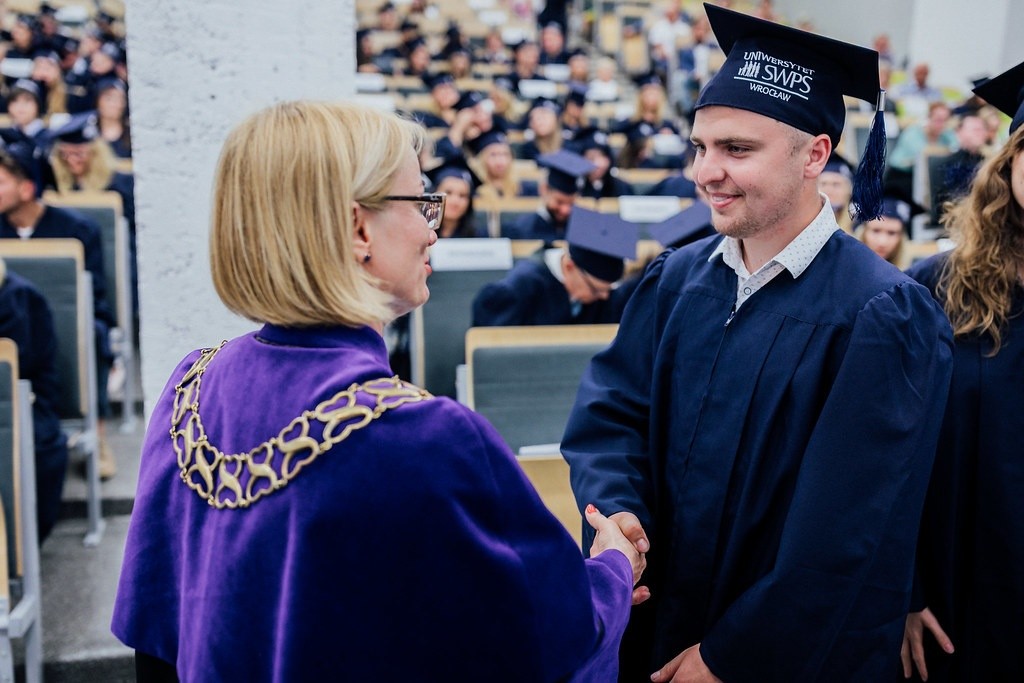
[{"xmin": 1, "ymin": 0, "xmax": 1012, "ymax": 683}]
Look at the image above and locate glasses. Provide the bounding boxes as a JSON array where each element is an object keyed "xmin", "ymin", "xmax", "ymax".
[
  {"xmin": 580, "ymin": 268, "xmax": 618, "ymax": 295},
  {"xmin": 363, "ymin": 190, "xmax": 450, "ymax": 231}
]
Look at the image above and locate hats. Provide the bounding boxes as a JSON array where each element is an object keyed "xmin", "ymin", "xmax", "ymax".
[
  {"xmin": 874, "ymin": 194, "xmax": 916, "ymax": 239},
  {"xmin": 822, "ymin": 151, "xmax": 856, "ymax": 181},
  {"xmin": 357, "ymin": 0, "xmax": 697, "ymax": 196},
  {"xmin": 0, "ymin": 3, "xmax": 129, "ymax": 202},
  {"xmin": 647, "ymin": 201, "xmax": 718, "ymax": 250},
  {"xmin": 971, "ymin": 61, "xmax": 1024, "ymax": 136},
  {"xmin": 951, "ymin": 95, "xmax": 986, "ymax": 121},
  {"xmin": 693, "ymin": 0, "xmax": 895, "ymax": 224},
  {"xmin": 567, "ymin": 204, "xmax": 636, "ymax": 283}
]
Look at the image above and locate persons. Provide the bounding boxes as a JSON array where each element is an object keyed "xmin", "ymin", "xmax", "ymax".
[
  {"xmin": 0, "ymin": 0, "xmax": 139, "ymax": 550},
  {"xmin": 889, "ymin": 59, "xmax": 1024, "ymax": 683},
  {"xmin": 559, "ymin": 0, "xmax": 956, "ymax": 683},
  {"xmin": 110, "ymin": 96, "xmax": 648, "ymax": 683},
  {"xmin": 353, "ymin": 0, "xmax": 1012, "ymax": 401}
]
